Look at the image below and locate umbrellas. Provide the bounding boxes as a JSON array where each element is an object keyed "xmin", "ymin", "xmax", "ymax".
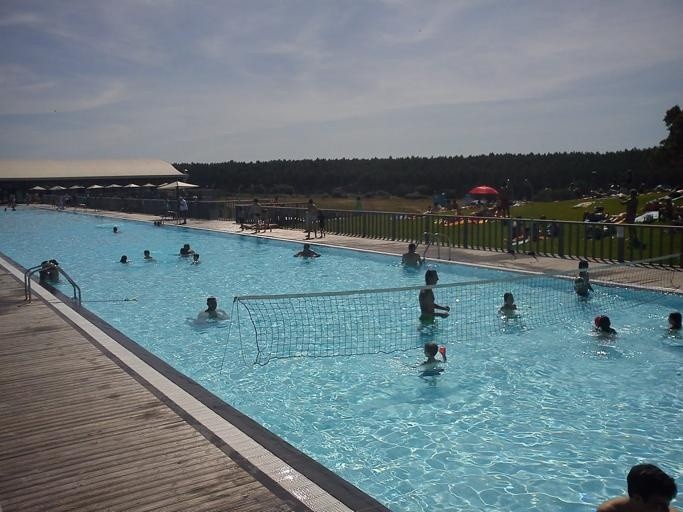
[
  {"xmin": 28, "ymin": 182, "xmax": 168, "ymax": 189},
  {"xmin": 156, "ymin": 181, "xmax": 197, "ymax": 220}
]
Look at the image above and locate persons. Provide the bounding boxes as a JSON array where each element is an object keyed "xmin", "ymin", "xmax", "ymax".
[
  {"xmin": 198, "ymin": 296, "xmax": 229, "ymax": 329},
  {"xmin": 294, "ymin": 244, "xmax": 322, "ymax": 261},
  {"xmin": 595, "ymin": 461, "xmax": 683, "ymax": 512},
  {"xmin": 576, "ymin": 261, "xmax": 595, "ymax": 303},
  {"xmin": 4, "ymin": 207, "xmax": 16, "ymax": 211},
  {"xmin": 113, "ymin": 227, "xmax": 118, "ymax": 233},
  {"xmin": 597, "ymin": 314, "xmax": 617, "ymax": 341},
  {"xmin": 661, "ymin": 311, "xmax": 682, "ymax": 340},
  {"xmin": 352, "ymin": 196, "xmax": 362, "ymax": 216},
  {"xmin": 416, "ymin": 267, "xmax": 450, "ymax": 340},
  {"xmin": 418, "ymin": 170, "xmax": 683, "ymax": 257},
  {"xmin": 143, "ymin": 250, "xmax": 152, "ymax": 259},
  {"xmin": 120, "ymin": 255, "xmax": 128, "ymax": 264},
  {"xmin": 180, "ymin": 244, "xmax": 201, "ymax": 265},
  {"xmin": 413, "ymin": 341, "xmax": 446, "ymax": 388},
  {"xmin": 400, "ymin": 244, "xmax": 426, "ymax": 272},
  {"xmin": 250, "ymin": 198, "xmax": 272, "ymax": 233},
  {"xmin": 497, "ymin": 291, "xmax": 517, "ymax": 316},
  {"xmin": 305, "ymin": 199, "xmax": 325, "ymax": 240},
  {"xmin": 238, "ymin": 207, "xmax": 245, "ymax": 231},
  {"xmin": 573, "ymin": 278, "xmax": 588, "ymax": 297}
]
